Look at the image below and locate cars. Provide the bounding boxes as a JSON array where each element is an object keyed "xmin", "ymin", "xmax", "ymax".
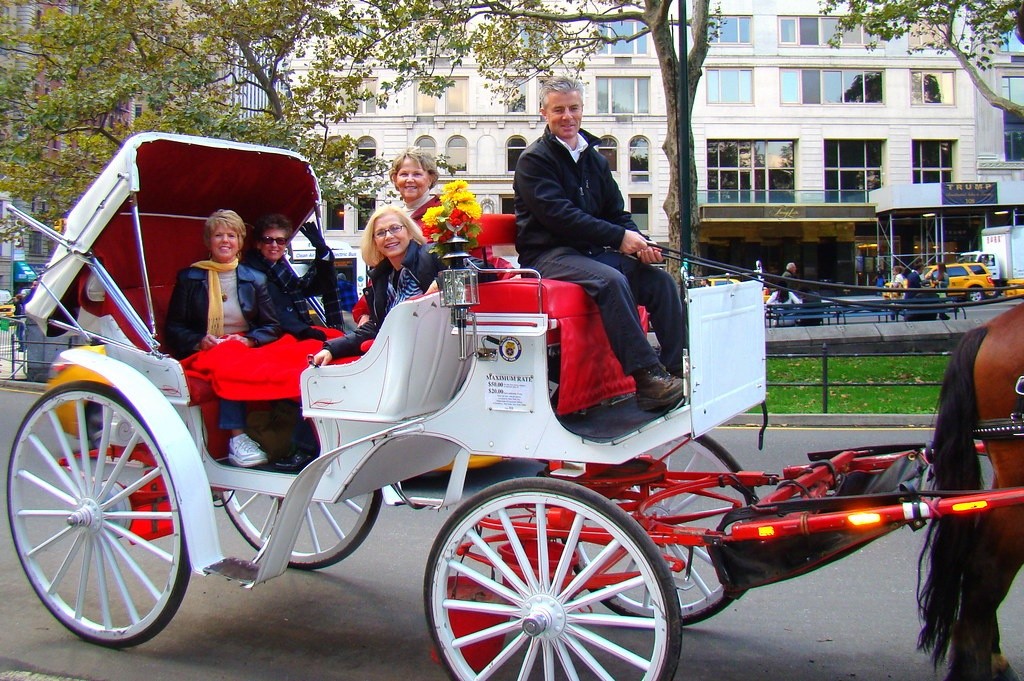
[
  {"xmin": 707, "ymin": 279, "xmax": 770, "ymax": 304},
  {"xmin": 1003, "ymin": 278, "xmax": 1024, "ymax": 298},
  {"xmin": 0, "ymin": 290, "xmax": 16, "ymax": 317}
]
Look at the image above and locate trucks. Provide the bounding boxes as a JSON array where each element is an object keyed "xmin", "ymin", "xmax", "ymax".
[{"xmin": 957, "ymin": 225, "xmax": 1024, "ymax": 282}]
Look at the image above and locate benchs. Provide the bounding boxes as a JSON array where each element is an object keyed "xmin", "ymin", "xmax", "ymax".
[
  {"xmin": 426, "ymin": 211, "xmax": 649, "ymax": 416},
  {"xmin": 765, "ymin": 295, "xmax": 960, "ymax": 328},
  {"xmin": 74, "ymin": 209, "xmax": 258, "ymax": 408}
]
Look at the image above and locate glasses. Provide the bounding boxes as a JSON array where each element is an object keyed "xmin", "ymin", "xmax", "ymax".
[
  {"xmin": 260, "ymin": 236, "xmax": 288, "ymax": 245},
  {"xmin": 374, "ymin": 224, "xmax": 404, "ymax": 238}
]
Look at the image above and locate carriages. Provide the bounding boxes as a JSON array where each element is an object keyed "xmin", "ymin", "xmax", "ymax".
[{"xmin": 9, "ymin": 130, "xmax": 1024, "ymax": 681}]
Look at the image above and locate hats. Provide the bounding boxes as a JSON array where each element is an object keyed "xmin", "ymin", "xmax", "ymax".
[{"xmin": 919, "ymin": 280, "xmax": 930, "ymax": 287}]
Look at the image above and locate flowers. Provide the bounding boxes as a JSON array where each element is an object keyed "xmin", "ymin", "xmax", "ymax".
[{"xmin": 419, "ymin": 177, "xmax": 484, "ymax": 258}]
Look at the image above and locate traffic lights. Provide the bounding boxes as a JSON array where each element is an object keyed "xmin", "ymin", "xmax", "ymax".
[{"xmin": 55, "ymin": 218, "xmax": 62, "ymax": 234}]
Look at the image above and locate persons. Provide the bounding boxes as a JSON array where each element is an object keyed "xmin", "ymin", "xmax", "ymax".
[
  {"xmin": 766, "ymin": 263, "xmax": 824, "ymax": 327},
  {"xmin": 163, "ymin": 146, "xmax": 498, "ymax": 470},
  {"xmin": 873, "ymin": 262, "xmax": 951, "ymax": 320},
  {"xmin": 11, "ymin": 280, "xmax": 39, "ymax": 350},
  {"xmin": 699, "ymin": 279, "xmax": 710, "ymax": 287},
  {"xmin": 513, "ymin": 75, "xmax": 690, "ymax": 410}
]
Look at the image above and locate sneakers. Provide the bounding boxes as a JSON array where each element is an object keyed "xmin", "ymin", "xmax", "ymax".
[{"xmin": 227, "ymin": 434, "xmax": 269, "ymax": 467}]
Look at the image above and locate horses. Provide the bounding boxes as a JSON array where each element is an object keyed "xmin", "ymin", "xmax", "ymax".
[{"xmin": 915, "ymin": 302, "xmax": 1023, "ymax": 681}]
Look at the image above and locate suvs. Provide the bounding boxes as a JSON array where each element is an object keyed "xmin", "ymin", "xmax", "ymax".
[{"xmin": 882, "ymin": 264, "xmax": 995, "ymax": 303}]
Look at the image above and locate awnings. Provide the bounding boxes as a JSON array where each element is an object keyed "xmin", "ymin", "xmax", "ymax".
[{"xmin": 13, "ymin": 262, "xmax": 46, "ymax": 282}]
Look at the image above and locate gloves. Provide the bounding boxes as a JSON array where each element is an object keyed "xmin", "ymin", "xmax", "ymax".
[
  {"xmin": 299, "ymin": 221, "xmax": 328, "ymax": 257},
  {"xmin": 299, "ymin": 328, "xmax": 326, "ymax": 341}
]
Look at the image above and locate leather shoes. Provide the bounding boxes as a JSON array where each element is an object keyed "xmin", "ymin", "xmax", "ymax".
[
  {"xmin": 638, "ymin": 366, "xmax": 684, "ymax": 410},
  {"xmin": 273, "ymin": 449, "xmax": 314, "ymax": 471}
]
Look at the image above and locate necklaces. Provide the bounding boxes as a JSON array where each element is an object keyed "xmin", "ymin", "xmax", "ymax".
[{"xmin": 220, "ymin": 271, "xmax": 232, "ymax": 301}]
